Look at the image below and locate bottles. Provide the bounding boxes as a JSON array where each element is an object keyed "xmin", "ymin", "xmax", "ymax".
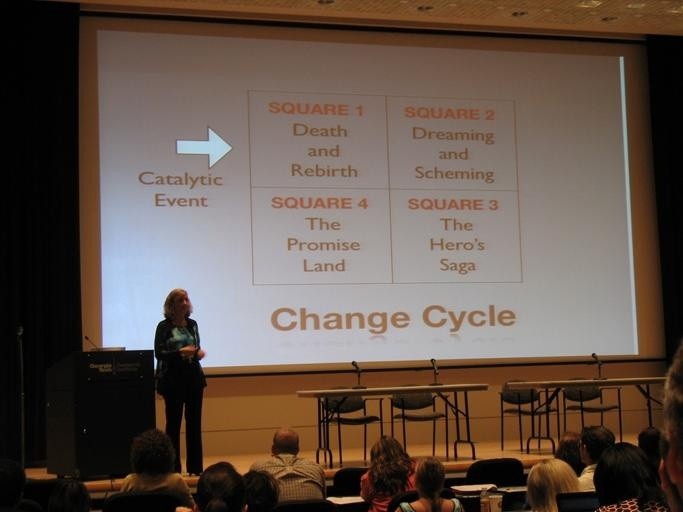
[{"xmin": 480, "ymin": 487, "xmax": 491, "ymax": 510}]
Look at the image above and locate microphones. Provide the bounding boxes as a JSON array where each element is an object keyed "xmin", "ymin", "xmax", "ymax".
[
  {"xmin": 592, "ymin": 353, "xmax": 602, "ymax": 365},
  {"xmin": 352, "ymin": 361, "xmax": 361, "ymax": 373},
  {"xmin": 85, "ymin": 336, "xmax": 99, "ymax": 351},
  {"xmin": 431, "ymin": 359, "xmax": 439, "ymax": 375}
]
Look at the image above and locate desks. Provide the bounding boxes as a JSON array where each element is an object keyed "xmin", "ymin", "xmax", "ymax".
[
  {"xmin": 501, "ymin": 376, "xmax": 667, "ymax": 451},
  {"xmin": 298, "ymin": 380, "xmax": 487, "ymax": 462}
]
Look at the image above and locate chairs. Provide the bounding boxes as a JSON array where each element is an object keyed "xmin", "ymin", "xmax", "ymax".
[
  {"xmin": 388, "ymin": 385, "xmax": 448, "ymax": 462},
  {"xmin": 314, "ymin": 386, "xmax": 380, "ymax": 461},
  {"xmin": 496, "ymin": 381, "xmax": 544, "ymax": 452},
  {"xmin": 562, "ymin": 374, "xmax": 619, "ymax": 452}
]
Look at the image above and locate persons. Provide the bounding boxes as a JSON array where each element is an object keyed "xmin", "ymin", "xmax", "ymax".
[
  {"xmin": 658, "ymin": 346, "xmax": 682, "ymax": 494},
  {"xmin": 152, "ymin": 288, "xmax": 206, "ymax": 476},
  {"xmin": 1, "ymin": 425, "xmax": 683, "ymax": 512}
]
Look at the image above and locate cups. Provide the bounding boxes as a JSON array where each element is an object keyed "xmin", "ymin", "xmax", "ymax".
[{"xmin": 489, "ymin": 493, "xmax": 504, "ymax": 511}]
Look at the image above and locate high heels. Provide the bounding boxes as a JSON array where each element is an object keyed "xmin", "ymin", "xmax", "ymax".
[{"xmin": 187, "ymin": 469, "xmax": 204, "ymax": 477}]
